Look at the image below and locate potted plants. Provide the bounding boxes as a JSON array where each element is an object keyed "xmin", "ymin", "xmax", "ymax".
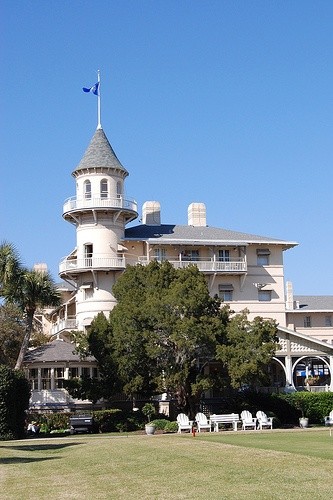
[
  {"xmin": 142, "ymin": 402, "xmax": 156, "ymax": 434},
  {"xmin": 291, "ymin": 391, "xmax": 316, "ymax": 429},
  {"xmin": 305, "ymin": 374, "xmax": 319, "ymax": 387}
]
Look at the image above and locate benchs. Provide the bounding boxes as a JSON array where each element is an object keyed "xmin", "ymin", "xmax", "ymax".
[{"xmin": 209, "ymin": 413, "xmax": 242, "ymax": 431}]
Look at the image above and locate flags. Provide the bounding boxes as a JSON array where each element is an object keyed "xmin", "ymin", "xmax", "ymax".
[{"xmin": 83, "ymin": 82, "xmax": 99, "ymax": 95}]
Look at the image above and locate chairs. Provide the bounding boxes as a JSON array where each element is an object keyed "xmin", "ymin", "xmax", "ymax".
[
  {"xmin": 240, "ymin": 409, "xmax": 258, "ymax": 430},
  {"xmin": 176, "ymin": 413, "xmax": 194, "ymax": 434},
  {"xmin": 324, "ymin": 410, "xmax": 333, "ymax": 426},
  {"xmin": 193, "ymin": 413, "xmax": 212, "ymax": 433},
  {"xmin": 256, "ymin": 411, "xmax": 274, "ymax": 430}
]
